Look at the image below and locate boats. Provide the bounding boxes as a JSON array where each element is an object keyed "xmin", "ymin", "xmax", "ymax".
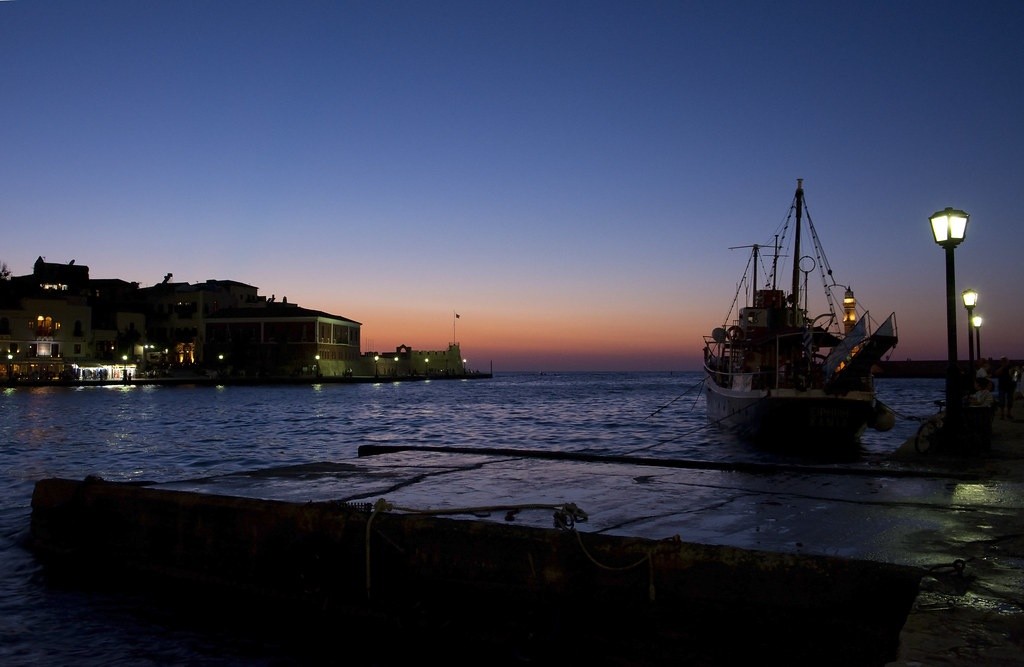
[{"xmin": 699, "ymin": 179, "xmax": 899, "ymax": 445}]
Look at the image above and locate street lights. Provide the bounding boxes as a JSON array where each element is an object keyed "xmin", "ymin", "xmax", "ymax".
[
  {"xmin": 394, "ymin": 356, "xmax": 400, "ymax": 374},
  {"xmin": 121, "ymin": 355, "xmax": 127, "ymax": 380},
  {"xmin": 314, "ymin": 355, "xmax": 321, "ymax": 376},
  {"xmin": 218, "ymin": 354, "xmax": 224, "ymax": 378},
  {"xmin": 463, "ymin": 360, "xmax": 466, "ymax": 373},
  {"xmin": 375, "ymin": 355, "xmax": 379, "ymax": 375},
  {"xmin": 963, "ymin": 289, "xmax": 983, "ymax": 357},
  {"xmin": 424, "ymin": 358, "xmax": 429, "ymax": 374},
  {"xmin": 929, "ymin": 207, "xmax": 970, "ymax": 365}
]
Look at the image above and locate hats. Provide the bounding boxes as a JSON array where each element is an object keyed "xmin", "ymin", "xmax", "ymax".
[{"xmin": 972, "ymin": 358, "xmax": 989, "ymax": 368}]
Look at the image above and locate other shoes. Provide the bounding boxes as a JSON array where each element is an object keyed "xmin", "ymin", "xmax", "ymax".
[
  {"xmin": 1006, "ymin": 414, "xmax": 1014, "ymax": 419},
  {"xmin": 999, "ymin": 416, "xmax": 1005, "ymax": 420}
]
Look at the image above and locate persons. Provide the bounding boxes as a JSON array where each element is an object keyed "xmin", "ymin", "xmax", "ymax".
[{"xmin": 977, "ymin": 356, "xmax": 1019, "ymax": 420}]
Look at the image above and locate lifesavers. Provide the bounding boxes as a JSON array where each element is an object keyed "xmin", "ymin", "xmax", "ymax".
[{"xmin": 727, "ymin": 325, "xmax": 744, "ymax": 342}]
